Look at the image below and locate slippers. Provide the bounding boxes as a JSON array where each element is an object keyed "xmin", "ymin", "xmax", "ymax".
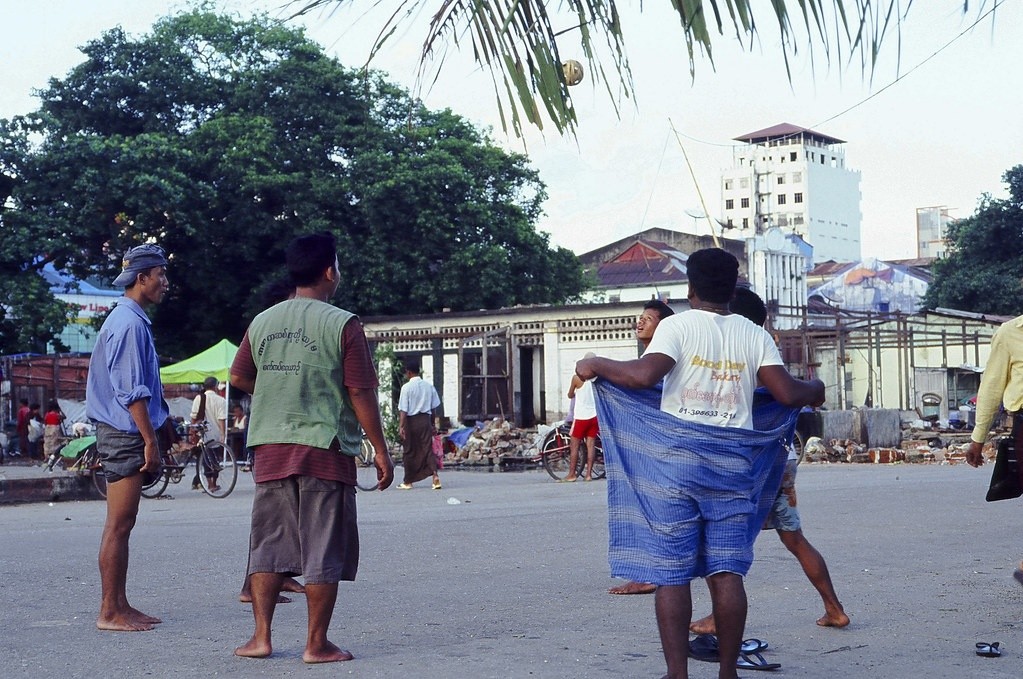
[
  {"xmin": 737, "ymin": 651, "xmax": 781, "ymax": 669},
  {"xmin": 740, "ymin": 638, "xmax": 768, "ymax": 654},
  {"xmin": 976, "ymin": 642, "xmax": 1001, "ymax": 657},
  {"xmin": 396, "ymin": 483, "xmax": 412, "ymax": 489},
  {"xmin": 431, "ymin": 484, "xmax": 442, "ymax": 490},
  {"xmin": 564, "ymin": 474, "xmax": 577, "ymax": 482},
  {"xmin": 583, "ymin": 476, "xmax": 592, "ymax": 481}
]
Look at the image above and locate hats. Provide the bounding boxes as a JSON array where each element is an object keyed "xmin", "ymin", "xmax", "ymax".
[
  {"xmin": 112, "ymin": 244, "xmax": 168, "ymax": 287},
  {"xmin": 584, "ymin": 352, "xmax": 596, "ymax": 358}
]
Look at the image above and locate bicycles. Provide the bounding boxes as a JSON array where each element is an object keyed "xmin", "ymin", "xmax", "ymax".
[
  {"xmin": 44, "ymin": 415, "xmax": 238, "ymax": 498},
  {"xmin": 353, "ymin": 427, "xmax": 382, "ymax": 492},
  {"xmin": 541, "ymin": 425, "xmax": 606, "ymax": 483}
]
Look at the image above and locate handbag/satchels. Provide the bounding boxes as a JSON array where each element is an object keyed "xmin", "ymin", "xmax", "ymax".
[
  {"xmin": 188, "ymin": 393, "xmax": 206, "ymax": 445},
  {"xmin": 986, "ymin": 436, "xmax": 1023, "ymax": 501}
]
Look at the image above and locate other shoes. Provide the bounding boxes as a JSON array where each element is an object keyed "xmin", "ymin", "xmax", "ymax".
[{"xmin": 687, "ymin": 633, "xmax": 719, "ymax": 662}]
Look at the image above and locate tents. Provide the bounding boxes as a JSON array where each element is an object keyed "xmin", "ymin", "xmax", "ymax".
[{"xmin": 160, "ymin": 338, "xmax": 239, "ymax": 471}]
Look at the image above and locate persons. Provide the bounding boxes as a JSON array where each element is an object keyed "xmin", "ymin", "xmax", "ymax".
[
  {"xmin": 240, "ymin": 529, "xmax": 306, "ymax": 604},
  {"xmin": 395, "ymin": 358, "xmax": 443, "ymax": 489},
  {"xmin": 16, "ymin": 398, "xmax": 66, "ymax": 462},
  {"xmin": 560, "ymin": 351, "xmax": 599, "ymax": 481},
  {"xmin": 689, "ymin": 290, "xmax": 849, "ymax": 634},
  {"xmin": 608, "ymin": 299, "xmax": 675, "ymax": 596},
  {"xmin": 966, "ymin": 312, "xmax": 1023, "ymax": 585},
  {"xmin": 83, "ymin": 244, "xmax": 170, "ymax": 632},
  {"xmin": 228, "ymin": 229, "xmax": 395, "ymax": 663},
  {"xmin": 156, "ymin": 376, "xmax": 254, "ymax": 493},
  {"xmin": 576, "ymin": 247, "xmax": 826, "ymax": 679}
]
